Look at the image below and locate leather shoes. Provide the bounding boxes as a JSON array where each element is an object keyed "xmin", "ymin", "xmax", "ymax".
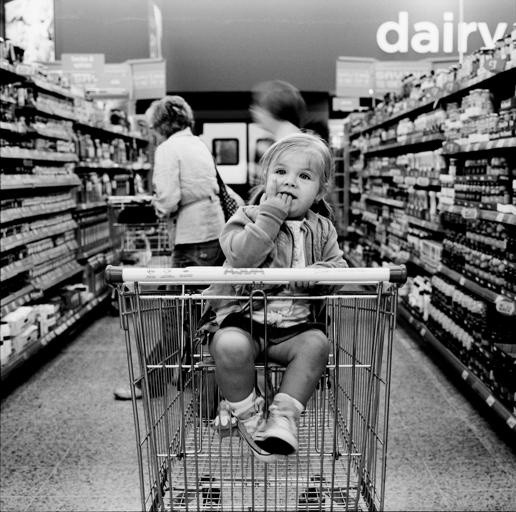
[{"xmin": 114, "ymin": 383, "xmax": 142, "ymax": 399}]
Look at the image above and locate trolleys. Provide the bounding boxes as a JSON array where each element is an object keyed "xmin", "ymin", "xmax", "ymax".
[
  {"xmin": 104, "ymin": 195, "xmax": 179, "ymax": 313},
  {"xmin": 104, "ymin": 264, "xmax": 408, "ymax": 512}
]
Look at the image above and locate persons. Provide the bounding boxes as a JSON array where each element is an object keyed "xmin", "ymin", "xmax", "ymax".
[
  {"xmin": 202, "ymin": 133, "xmax": 353, "ymax": 462},
  {"xmin": 112, "ymin": 94, "xmax": 227, "ymax": 399},
  {"xmin": 250, "ymin": 81, "xmax": 311, "ymax": 141}
]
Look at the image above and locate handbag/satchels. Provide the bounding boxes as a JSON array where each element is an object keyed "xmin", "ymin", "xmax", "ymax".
[{"xmin": 216, "ymin": 169, "xmax": 239, "ymax": 222}]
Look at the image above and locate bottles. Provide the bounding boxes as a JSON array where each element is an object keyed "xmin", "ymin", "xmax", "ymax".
[
  {"xmin": 76, "ymin": 136, "xmax": 140, "ymax": 301},
  {"xmin": 431, "ymin": 149, "xmax": 516, "ymax": 415}
]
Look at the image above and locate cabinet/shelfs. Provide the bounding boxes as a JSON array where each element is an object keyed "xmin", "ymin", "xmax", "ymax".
[
  {"xmin": 343, "ymin": 60, "xmax": 515, "ymax": 455},
  {"xmin": 1, "ymin": 58, "xmax": 155, "ymax": 403}
]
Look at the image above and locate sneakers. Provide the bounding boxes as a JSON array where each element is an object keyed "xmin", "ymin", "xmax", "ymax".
[{"xmin": 230, "ymin": 397, "xmax": 299, "ymax": 461}]
